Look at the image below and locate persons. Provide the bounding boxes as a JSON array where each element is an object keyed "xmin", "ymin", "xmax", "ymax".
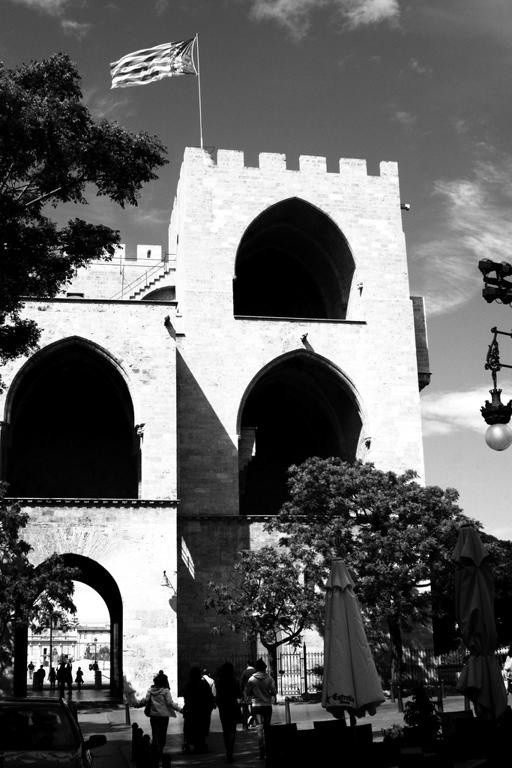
[
  {"xmin": 93, "ymin": 661, "xmax": 98, "ymax": 672},
  {"xmin": 28, "ymin": 658, "xmax": 84, "ymax": 701},
  {"xmin": 133, "ymin": 657, "xmax": 277, "ymax": 767}
]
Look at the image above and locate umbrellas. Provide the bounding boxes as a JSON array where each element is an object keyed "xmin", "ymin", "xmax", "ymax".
[
  {"xmin": 321, "ymin": 557, "xmax": 387, "ymax": 727},
  {"xmin": 457, "ymin": 522, "xmax": 508, "ymax": 724}
]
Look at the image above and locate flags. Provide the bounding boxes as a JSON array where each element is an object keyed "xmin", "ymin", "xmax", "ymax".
[{"xmin": 109, "ymin": 37, "xmax": 198, "ymax": 89}]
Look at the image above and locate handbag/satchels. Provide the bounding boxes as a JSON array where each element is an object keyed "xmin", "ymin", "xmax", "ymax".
[{"xmin": 143, "ymin": 700, "xmax": 152, "ymax": 718}]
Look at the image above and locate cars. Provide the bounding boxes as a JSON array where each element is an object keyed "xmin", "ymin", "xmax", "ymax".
[{"xmin": 0, "ymin": 696, "xmax": 108, "ymax": 768}]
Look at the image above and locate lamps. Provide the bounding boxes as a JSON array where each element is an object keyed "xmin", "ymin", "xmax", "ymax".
[
  {"xmin": 161, "ymin": 570, "xmax": 177, "ymax": 595},
  {"xmin": 480, "ymin": 326, "xmax": 512, "ymax": 451}
]
